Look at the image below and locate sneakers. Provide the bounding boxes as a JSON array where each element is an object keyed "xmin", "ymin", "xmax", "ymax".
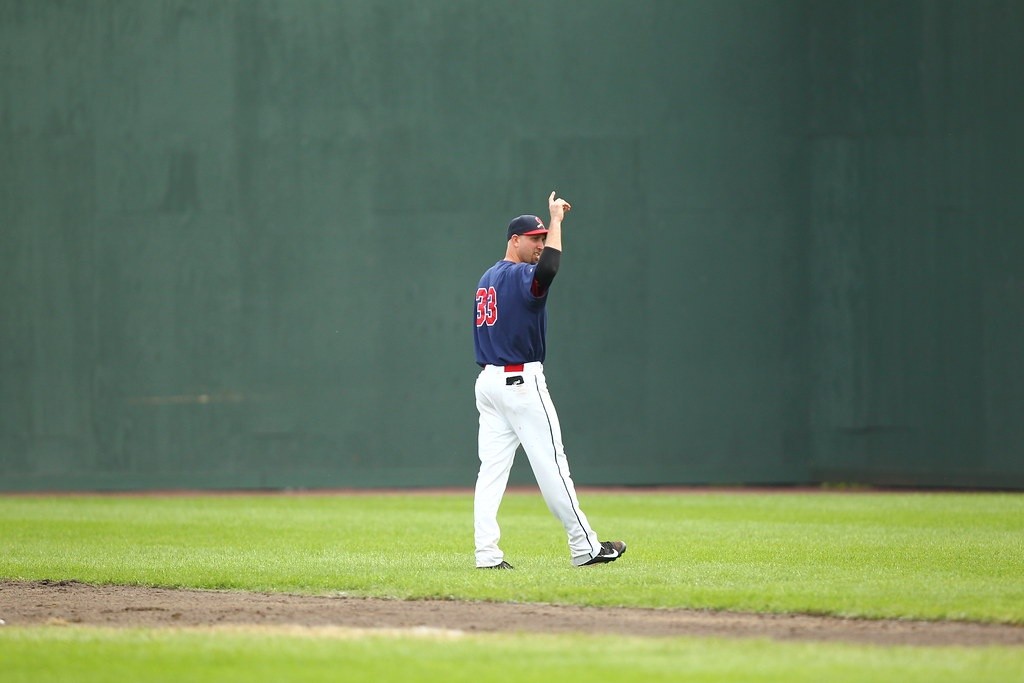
[
  {"xmin": 477, "ymin": 560, "xmax": 513, "ymax": 570},
  {"xmin": 578, "ymin": 541, "xmax": 626, "ymax": 567}
]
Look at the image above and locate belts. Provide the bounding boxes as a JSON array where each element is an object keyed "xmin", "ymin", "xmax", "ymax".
[{"xmin": 504, "ymin": 364, "xmax": 524, "ymax": 372}]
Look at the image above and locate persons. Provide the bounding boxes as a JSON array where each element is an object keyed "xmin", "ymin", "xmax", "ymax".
[{"xmin": 472, "ymin": 191, "xmax": 627, "ymax": 569}]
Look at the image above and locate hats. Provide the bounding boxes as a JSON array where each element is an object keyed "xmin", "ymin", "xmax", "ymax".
[{"xmin": 507, "ymin": 214, "xmax": 548, "ymax": 241}]
